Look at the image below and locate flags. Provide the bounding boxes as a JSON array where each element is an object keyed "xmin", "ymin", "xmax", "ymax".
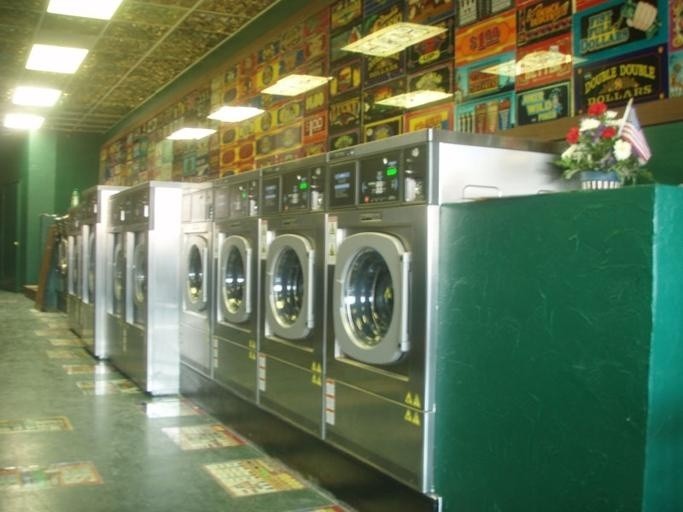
[{"xmin": 622, "ymin": 100, "xmax": 652, "ymax": 162}]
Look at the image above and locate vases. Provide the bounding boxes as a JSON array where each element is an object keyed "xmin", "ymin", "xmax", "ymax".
[{"xmin": 580, "ymin": 168, "xmax": 625, "ymax": 190}]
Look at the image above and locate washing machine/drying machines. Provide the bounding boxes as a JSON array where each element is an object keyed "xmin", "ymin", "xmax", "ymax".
[
  {"xmin": 181, "ymin": 127, "xmax": 581, "ymax": 498},
  {"xmin": 109, "ymin": 182, "xmax": 180, "ymax": 398},
  {"xmin": 56, "ymin": 185, "xmax": 129, "ymax": 361}
]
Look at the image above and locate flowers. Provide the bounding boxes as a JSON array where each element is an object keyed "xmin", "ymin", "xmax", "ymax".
[{"xmin": 546, "ymin": 100, "xmax": 656, "ymax": 181}]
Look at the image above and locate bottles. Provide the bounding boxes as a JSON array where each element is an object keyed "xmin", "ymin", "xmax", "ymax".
[
  {"xmin": 70, "ymin": 188, "xmax": 79, "ymax": 208},
  {"xmin": 346, "ymin": 25, "xmax": 362, "ymax": 57},
  {"xmin": 459, "ymin": 98, "xmax": 512, "ymax": 135}
]
